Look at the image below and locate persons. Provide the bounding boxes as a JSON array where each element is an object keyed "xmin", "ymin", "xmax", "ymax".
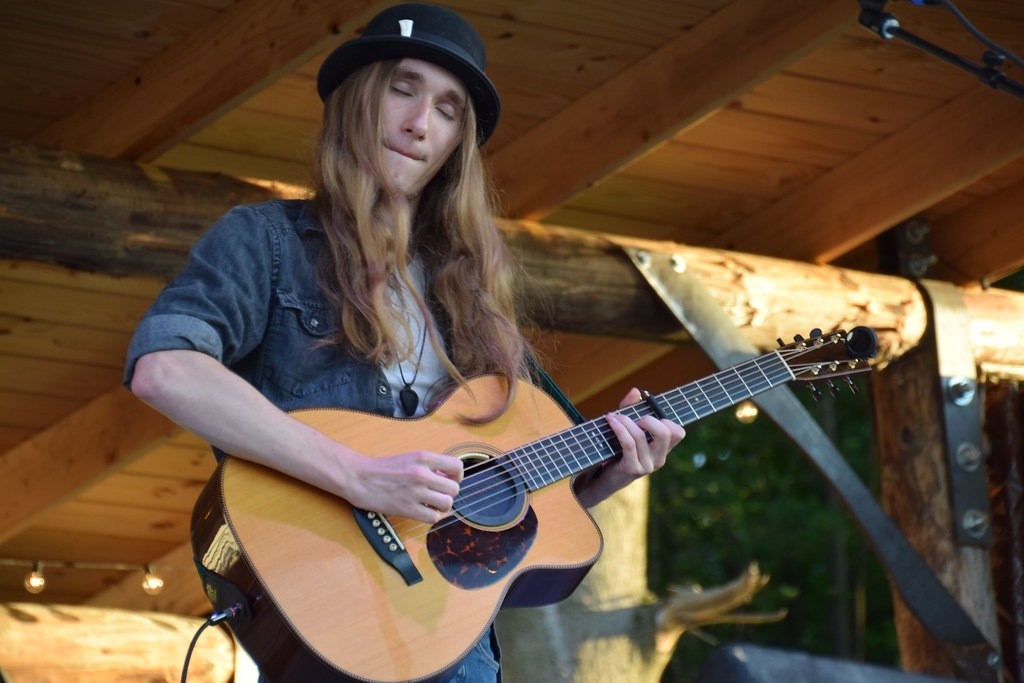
[{"xmin": 123, "ymin": 1, "xmax": 690, "ymax": 683}]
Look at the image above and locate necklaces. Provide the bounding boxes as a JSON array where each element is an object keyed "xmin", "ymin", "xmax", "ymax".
[{"xmin": 395, "ymin": 268, "xmax": 428, "ymax": 417}]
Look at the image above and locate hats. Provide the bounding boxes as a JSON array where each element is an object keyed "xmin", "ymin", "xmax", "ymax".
[{"xmin": 315, "ymin": 4, "xmax": 500, "ymax": 148}]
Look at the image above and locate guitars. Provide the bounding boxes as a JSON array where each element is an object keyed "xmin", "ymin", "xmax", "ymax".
[{"xmin": 188, "ymin": 325, "xmax": 880, "ymax": 682}]
